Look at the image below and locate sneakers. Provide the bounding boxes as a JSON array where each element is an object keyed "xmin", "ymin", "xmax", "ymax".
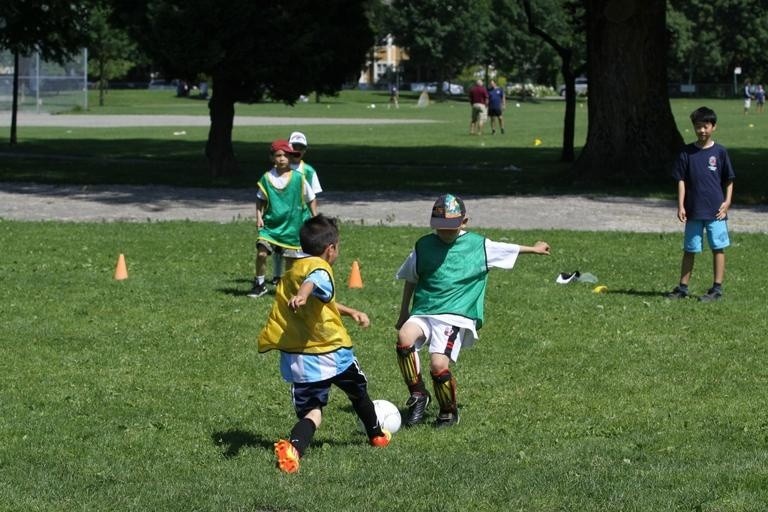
[
  {"xmin": 434, "ymin": 408, "xmax": 461, "ymax": 430},
  {"xmin": 406, "ymin": 389, "xmax": 431, "ymax": 428},
  {"xmin": 273, "ymin": 439, "xmax": 300, "ymax": 474},
  {"xmin": 372, "ymin": 432, "xmax": 392, "ymax": 447},
  {"xmin": 247, "ymin": 276, "xmax": 281, "ymax": 298},
  {"xmin": 698, "ymin": 288, "xmax": 724, "ymax": 301},
  {"xmin": 668, "ymin": 286, "xmax": 690, "ymax": 299}
]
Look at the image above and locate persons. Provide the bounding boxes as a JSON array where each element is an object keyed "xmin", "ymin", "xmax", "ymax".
[
  {"xmin": 741, "ymin": 78, "xmax": 754, "ymax": 115},
  {"xmin": 466, "ymin": 78, "xmax": 488, "ymax": 133},
  {"xmin": 246, "ymin": 138, "xmax": 318, "ymax": 299},
  {"xmin": 754, "ymin": 83, "xmax": 765, "ymax": 114},
  {"xmin": 271, "ymin": 130, "xmax": 323, "ymax": 287},
  {"xmin": 665, "ymin": 105, "xmax": 735, "ymax": 304},
  {"xmin": 486, "ymin": 80, "xmax": 506, "ymax": 134},
  {"xmin": 393, "ymin": 193, "xmax": 551, "ymax": 431},
  {"xmin": 386, "ymin": 83, "xmax": 400, "ymax": 111},
  {"xmin": 256, "ymin": 214, "xmax": 392, "ymax": 476}
]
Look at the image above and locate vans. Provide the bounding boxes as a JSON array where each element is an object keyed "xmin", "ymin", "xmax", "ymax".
[{"xmin": 149, "ymin": 78, "xmax": 180, "ymax": 91}]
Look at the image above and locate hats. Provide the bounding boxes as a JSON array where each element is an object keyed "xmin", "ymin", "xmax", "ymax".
[
  {"xmin": 556, "ymin": 271, "xmax": 598, "ymax": 287},
  {"xmin": 430, "ymin": 194, "xmax": 466, "ymax": 230},
  {"xmin": 272, "ymin": 140, "xmax": 301, "ymax": 158},
  {"xmin": 288, "ymin": 132, "xmax": 307, "ymax": 146}
]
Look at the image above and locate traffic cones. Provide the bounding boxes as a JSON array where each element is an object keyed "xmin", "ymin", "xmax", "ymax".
[
  {"xmin": 347, "ymin": 260, "xmax": 368, "ymax": 290},
  {"xmin": 533, "ymin": 138, "xmax": 542, "ymax": 147},
  {"xmin": 113, "ymin": 253, "xmax": 129, "ymax": 283}
]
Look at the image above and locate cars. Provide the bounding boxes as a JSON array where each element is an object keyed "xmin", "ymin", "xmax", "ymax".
[
  {"xmin": 42, "ymin": 73, "xmax": 84, "ymax": 93},
  {"xmin": 558, "ymin": 77, "xmax": 587, "ymax": 97},
  {"xmin": 419, "ymin": 81, "xmax": 462, "ymax": 96}
]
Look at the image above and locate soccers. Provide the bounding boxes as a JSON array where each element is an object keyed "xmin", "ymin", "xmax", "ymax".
[{"xmin": 359, "ymin": 398, "xmax": 402, "ymax": 434}]
